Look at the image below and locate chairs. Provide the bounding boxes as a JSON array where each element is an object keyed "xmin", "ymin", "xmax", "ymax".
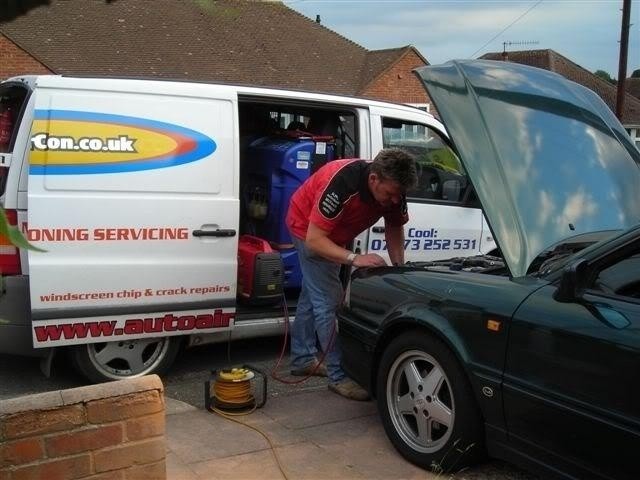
[
  {"xmin": 291, "ymin": 360, "xmax": 328, "ymax": 376},
  {"xmin": 328, "ymin": 375, "xmax": 369, "ymax": 400}
]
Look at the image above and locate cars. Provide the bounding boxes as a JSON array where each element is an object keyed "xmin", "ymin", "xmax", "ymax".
[{"xmin": 337, "ymin": 59, "xmax": 640, "ymax": 480}]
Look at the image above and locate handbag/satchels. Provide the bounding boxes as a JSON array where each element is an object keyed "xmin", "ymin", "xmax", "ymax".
[{"xmin": 346, "ymin": 252, "xmax": 358, "ymax": 266}]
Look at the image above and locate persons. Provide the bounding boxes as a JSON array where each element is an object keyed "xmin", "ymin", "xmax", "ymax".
[{"xmin": 285, "ymin": 148, "xmax": 418, "ymax": 401}]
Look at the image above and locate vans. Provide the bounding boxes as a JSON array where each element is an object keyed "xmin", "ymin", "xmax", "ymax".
[{"xmin": 0, "ymin": 75, "xmax": 501, "ymax": 387}]
